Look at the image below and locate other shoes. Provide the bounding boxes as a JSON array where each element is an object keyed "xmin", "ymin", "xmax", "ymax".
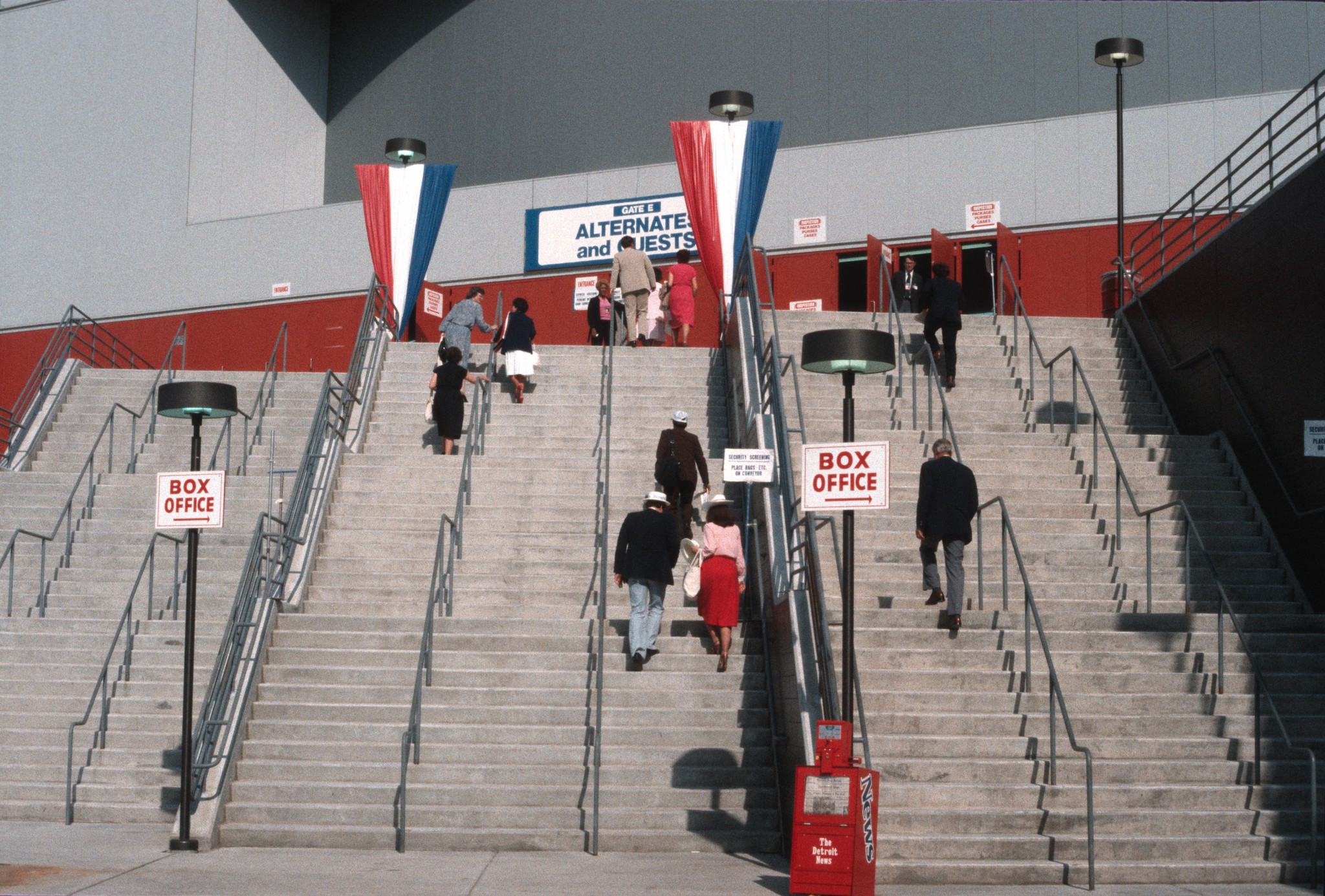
[
  {"xmin": 637, "ymin": 333, "xmax": 649, "ymax": 347},
  {"xmin": 625, "ymin": 340, "xmax": 636, "ymax": 348},
  {"xmin": 681, "ymin": 341, "xmax": 688, "ymax": 347},
  {"xmin": 646, "ymin": 648, "xmax": 660, "ymax": 655},
  {"xmin": 633, "ymin": 653, "xmax": 643, "ymax": 672}
]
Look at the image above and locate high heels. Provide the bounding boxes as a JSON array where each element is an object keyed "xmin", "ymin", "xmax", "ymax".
[
  {"xmin": 717, "ymin": 653, "xmax": 728, "ymax": 672},
  {"xmin": 514, "ymin": 384, "xmax": 522, "ymax": 398},
  {"xmin": 516, "ymin": 396, "xmax": 523, "ymax": 403},
  {"xmin": 711, "ymin": 640, "xmax": 721, "ymax": 655}
]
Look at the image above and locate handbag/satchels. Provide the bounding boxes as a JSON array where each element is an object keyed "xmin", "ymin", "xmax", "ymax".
[
  {"xmin": 438, "ymin": 337, "xmax": 448, "ymax": 364},
  {"xmin": 492, "ymin": 311, "xmax": 510, "ymax": 351},
  {"xmin": 657, "ymin": 430, "xmax": 680, "ymax": 485},
  {"xmin": 914, "ymin": 311, "xmax": 927, "ymax": 324},
  {"xmin": 424, "ymin": 389, "xmax": 436, "ymax": 424},
  {"xmin": 682, "ymin": 548, "xmax": 704, "ymax": 601}
]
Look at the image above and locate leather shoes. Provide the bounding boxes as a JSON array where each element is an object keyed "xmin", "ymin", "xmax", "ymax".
[
  {"xmin": 929, "ymin": 351, "xmax": 942, "ymax": 365},
  {"xmin": 947, "ymin": 376, "xmax": 955, "ymax": 387},
  {"xmin": 926, "ymin": 592, "xmax": 945, "ymax": 606},
  {"xmin": 944, "ymin": 615, "xmax": 961, "ymax": 628}
]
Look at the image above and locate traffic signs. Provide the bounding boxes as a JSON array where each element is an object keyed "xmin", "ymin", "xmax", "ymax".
[
  {"xmin": 801, "ymin": 442, "xmax": 890, "ymax": 509},
  {"xmin": 154, "ymin": 471, "xmax": 225, "ymax": 529}
]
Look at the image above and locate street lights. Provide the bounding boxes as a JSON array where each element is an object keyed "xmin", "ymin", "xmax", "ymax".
[
  {"xmin": 1094, "ymin": 36, "xmax": 1148, "ymax": 313},
  {"xmin": 155, "ymin": 381, "xmax": 237, "ymax": 850},
  {"xmin": 798, "ymin": 327, "xmax": 899, "ymax": 758}
]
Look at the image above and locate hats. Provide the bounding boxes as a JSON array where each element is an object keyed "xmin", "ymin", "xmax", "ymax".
[
  {"xmin": 642, "ymin": 491, "xmax": 670, "ymax": 506},
  {"xmin": 670, "ymin": 411, "xmax": 689, "ymax": 423},
  {"xmin": 702, "ymin": 494, "xmax": 734, "ymax": 509},
  {"xmin": 680, "ymin": 538, "xmax": 700, "ymax": 565}
]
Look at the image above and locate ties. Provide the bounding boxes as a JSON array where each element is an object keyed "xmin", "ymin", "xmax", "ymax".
[{"xmin": 904, "ymin": 272, "xmax": 911, "ymax": 300}]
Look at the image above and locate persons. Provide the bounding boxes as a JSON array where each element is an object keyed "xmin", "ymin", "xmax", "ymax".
[
  {"xmin": 917, "ymin": 439, "xmax": 978, "ymax": 628},
  {"xmin": 893, "ymin": 254, "xmax": 965, "ymax": 387},
  {"xmin": 428, "ymin": 236, "xmax": 696, "ymax": 456},
  {"xmin": 692, "ymin": 495, "xmax": 745, "ymax": 672},
  {"xmin": 653, "ymin": 410, "xmax": 712, "ymax": 545},
  {"xmin": 614, "ymin": 492, "xmax": 681, "ymax": 673}
]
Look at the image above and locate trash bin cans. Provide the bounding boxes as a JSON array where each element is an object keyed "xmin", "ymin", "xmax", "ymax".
[
  {"xmin": 1100, "ymin": 269, "xmax": 1143, "ymax": 317},
  {"xmin": 792, "ymin": 718, "xmax": 880, "ymax": 895}
]
